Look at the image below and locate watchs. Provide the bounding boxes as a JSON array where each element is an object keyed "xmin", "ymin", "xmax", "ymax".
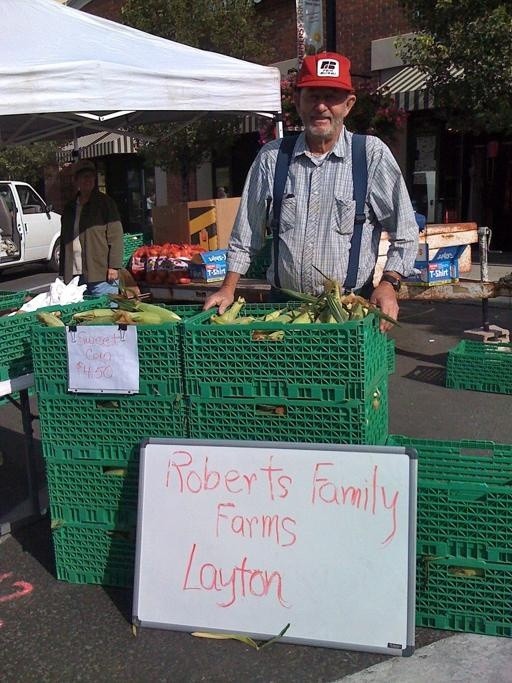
[{"xmin": 379, "ymin": 271, "xmax": 402, "ymax": 292}]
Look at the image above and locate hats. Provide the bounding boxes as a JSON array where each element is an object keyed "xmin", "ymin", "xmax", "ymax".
[
  {"xmin": 73, "ymin": 160, "xmax": 95, "ymax": 177},
  {"xmin": 296, "ymin": 51, "xmax": 352, "ymax": 92}
]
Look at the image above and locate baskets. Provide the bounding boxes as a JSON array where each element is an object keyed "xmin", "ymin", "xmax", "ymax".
[
  {"xmin": 53, "ymin": 528, "xmax": 137, "ymax": 586},
  {"xmin": 46, "ymin": 460, "xmax": 140, "ymax": 530},
  {"xmin": 37, "ymin": 396, "xmax": 189, "ymax": 462},
  {"xmin": 182, "ymin": 303, "xmax": 387, "ymax": 405},
  {"xmin": 188, "ymin": 403, "xmax": 389, "ymax": 447}
]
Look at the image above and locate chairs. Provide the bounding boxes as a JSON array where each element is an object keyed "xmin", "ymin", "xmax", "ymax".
[{"xmin": 0, "ymin": 196, "xmax": 17, "ymax": 236}]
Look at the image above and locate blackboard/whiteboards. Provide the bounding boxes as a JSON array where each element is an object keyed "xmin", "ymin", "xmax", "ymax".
[{"xmin": 132, "ymin": 436, "xmax": 418, "ymax": 658}]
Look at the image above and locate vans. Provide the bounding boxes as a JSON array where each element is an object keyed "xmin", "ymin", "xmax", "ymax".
[{"xmin": 1, "ymin": 180, "xmax": 63, "ymax": 277}]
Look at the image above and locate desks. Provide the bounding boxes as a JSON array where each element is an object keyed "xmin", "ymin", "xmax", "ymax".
[
  {"xmin": 1, "ymin": 373, "xmax": 44, "ymax": 508},
  {"xmin": 134, "ymin": 277, "xmax": 272, "ymax": 303}
]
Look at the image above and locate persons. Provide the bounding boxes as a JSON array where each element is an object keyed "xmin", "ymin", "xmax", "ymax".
[
  {"xmin": 55, "ymin": 163, "xmax": 126, "ymax": 309},
  {"xmin": 199, "ymin": 48, "xmax": 422, "ymax": 335},
  {"xmin": 410, "ymin": 197, "xmax": 426, "ymax": 232}
]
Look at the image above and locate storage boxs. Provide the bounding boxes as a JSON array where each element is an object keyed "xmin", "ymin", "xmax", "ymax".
[
  {"xmin": 399, "ymin": 243, "xmax": 467, "ymax": 287},
  {"xmin": 188, "ymin": 249, "xmax": 230, "ymax": 283}
]
